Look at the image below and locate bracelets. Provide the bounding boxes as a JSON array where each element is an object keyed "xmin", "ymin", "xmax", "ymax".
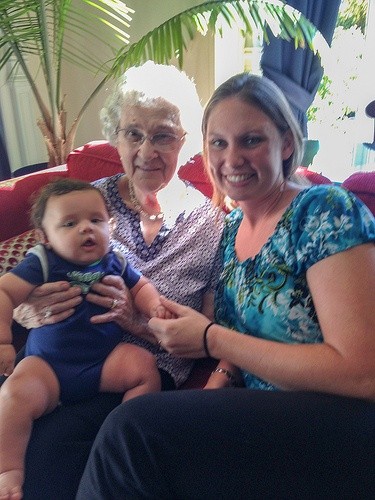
[
  {"xmin": 203, "ymin": 322, "xmax": 217, "ymax": 358},
  {"xmin": 212, "ymin": 367, "xmax": 235, "ymax": 386}
]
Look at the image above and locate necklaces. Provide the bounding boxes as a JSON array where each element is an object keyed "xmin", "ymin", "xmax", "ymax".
[{"xmin": 128, "ymin": 180, "xmax": 190, "ymax": 221}]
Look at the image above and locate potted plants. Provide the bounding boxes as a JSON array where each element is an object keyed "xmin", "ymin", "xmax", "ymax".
[{"xmin": 0, "ymin": 0, "xmax": 331, "ymax": 180}]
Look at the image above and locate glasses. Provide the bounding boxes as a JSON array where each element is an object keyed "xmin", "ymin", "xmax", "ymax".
[{"xmin": 114, "ymin": 117, "xmax": 187, "ymax": 149}]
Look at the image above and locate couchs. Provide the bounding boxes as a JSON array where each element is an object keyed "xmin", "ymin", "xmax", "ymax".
[{"xmin": 0, "ymin": 140, "xmax": 375, "ymax": 278}]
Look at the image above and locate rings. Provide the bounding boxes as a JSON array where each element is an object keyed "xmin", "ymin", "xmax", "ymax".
[
  {"xmin": 158, "ymin": 339, "xmax": 161, "ymax": 343},
  {"xmin": 43, "ymin": 306, "xmax": 53, "ymax": 318},
  {"xmin": 111, "ymin": 298, "xmax": 118, "ymax": 310}
]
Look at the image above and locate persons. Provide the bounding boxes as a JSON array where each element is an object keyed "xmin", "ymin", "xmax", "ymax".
[
  {"xmin": 0, "ymin": 179, "xmax": 176, "ymax": 500},
  {"xmin": 0, "ymin": 60, "xmax": 226, "ymax": 500},
  {"xmin": 77, "ymin": 74, "xmax": 375, "ymax": 500}
]
